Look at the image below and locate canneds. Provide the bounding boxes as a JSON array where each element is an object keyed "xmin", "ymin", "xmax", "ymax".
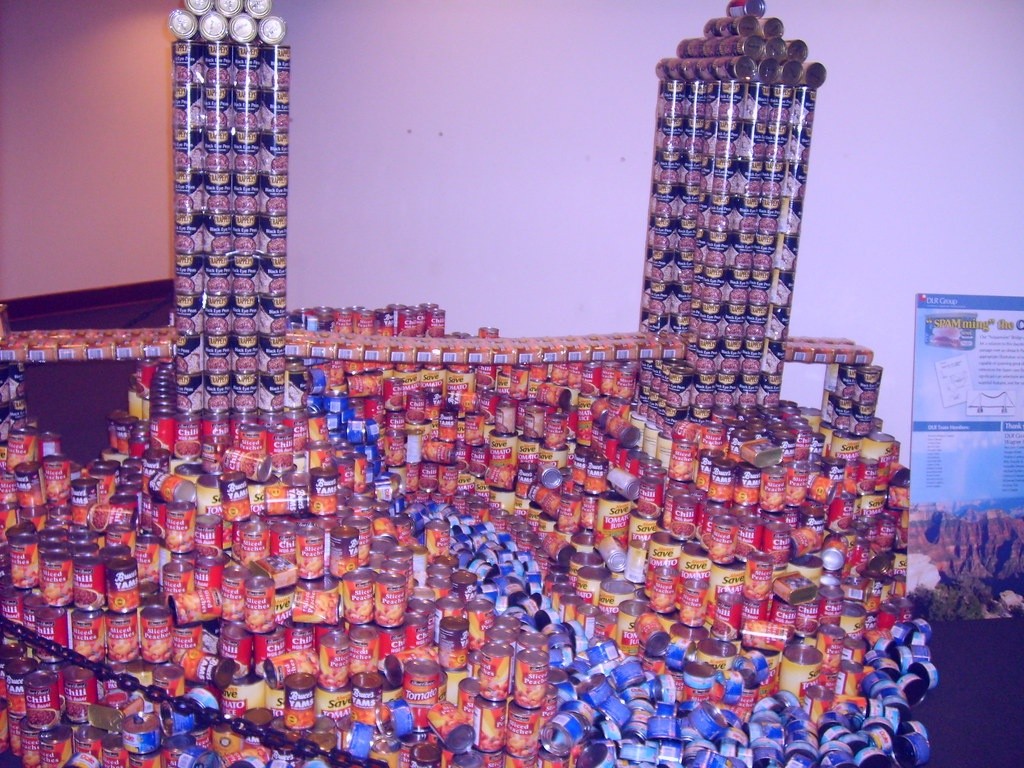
[{"xmin": 0, "ymin": 0, "xmax": 939, "ymax": 768}]
